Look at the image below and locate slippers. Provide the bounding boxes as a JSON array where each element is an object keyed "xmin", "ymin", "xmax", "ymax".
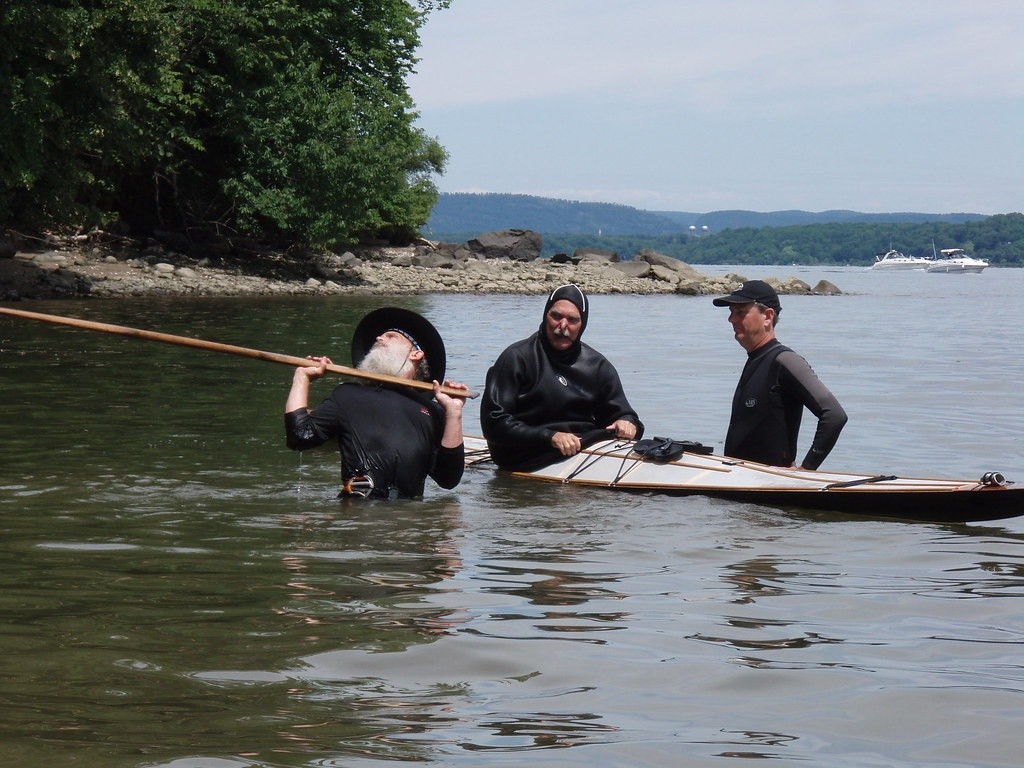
[{"xmin": 634, "ymin": 436, "xmax": 714, "ymax": 463}]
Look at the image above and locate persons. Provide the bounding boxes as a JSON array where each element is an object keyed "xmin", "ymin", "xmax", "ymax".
[
  {"xmin": 480, "ymin": 284, "xmax": 645, "ymax": 471},
  {"xmin": 713, "ymin": 280, "xmax": 848, "ymax": 471},
  {"xmin": 284, "ymin": 307, "xmax": 468, "ymax": 501}
]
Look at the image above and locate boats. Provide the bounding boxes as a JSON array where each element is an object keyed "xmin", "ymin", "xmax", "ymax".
[
  {"xmin": 462, "ymin": 434, "xmax": 1024, "ymax": 522},
  {"xmin": 871, "ymin": 250, "xmax": 930, "ymax": 270},
  {"xmin": 927, "ymin": 248, "xmax": 989, "ymax": 273}
]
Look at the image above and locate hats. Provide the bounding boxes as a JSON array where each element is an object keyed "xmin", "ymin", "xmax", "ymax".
[
  {"xmin": 350, "ymin": 307, "xmax": 446, "ymax": 386},
  {"xmin": 713, "ymin": 280, "xmax": 782, "ymax": 316}
]
